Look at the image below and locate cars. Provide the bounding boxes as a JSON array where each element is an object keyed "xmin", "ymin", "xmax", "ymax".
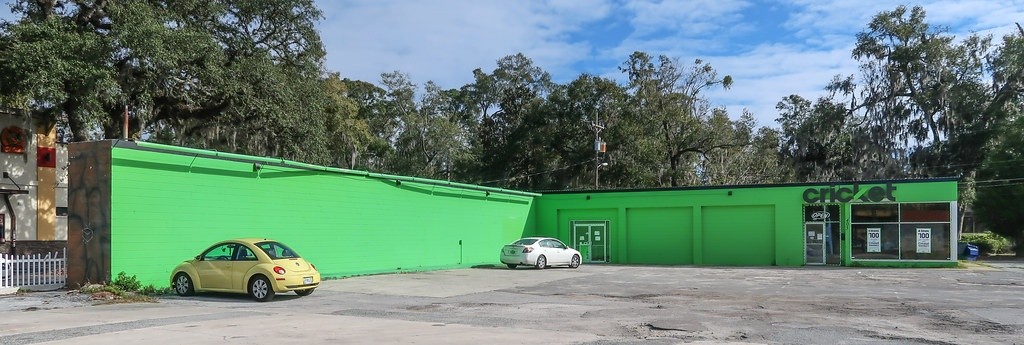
[
  {"xmin": 499, "ymin": 236, "xmax": 582, "ymax": 269},
  {"xmin": 2, "ymin": 258, "xmax": 10, "ymax": 281},
  {"xmin": 170, "ymin": 238, "xmax": 321, "ymax": 302}
]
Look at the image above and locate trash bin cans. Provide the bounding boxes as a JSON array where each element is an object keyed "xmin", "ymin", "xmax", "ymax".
[{"xmin": 957, "ymin": 240, "xmax": 979, "ymax": 261}]
